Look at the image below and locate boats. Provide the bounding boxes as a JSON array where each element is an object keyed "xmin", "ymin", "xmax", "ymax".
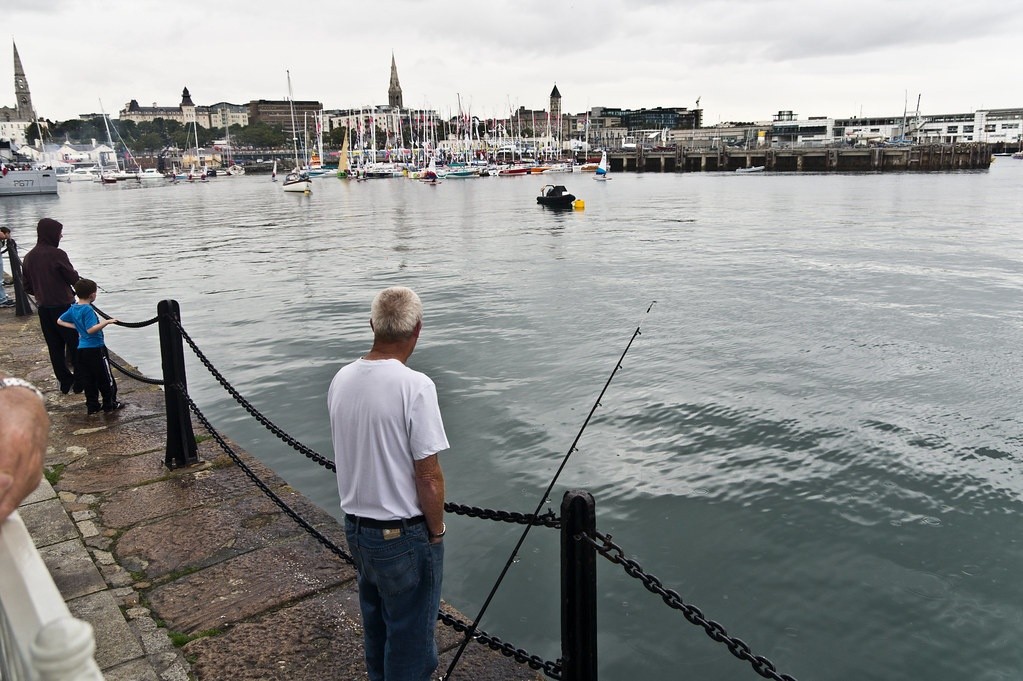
[
  {"xmin": 736, "ymin": 164, "xmax": 765, "ymax": 173},
  {"xmin": 537, "ymin": 185, "xmax": 575, "ymax": 207}
]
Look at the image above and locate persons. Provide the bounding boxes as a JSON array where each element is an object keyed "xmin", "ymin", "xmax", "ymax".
[
  {"xmin": 57, "ymin": 279, "xmax": 125, "ymax": 415},
  {"xmin": 0, "ymin": 373, "xmax": 49, "ymax": 520},
  {"xmin": 326, "ymin": 286, "xmax": 450, "ymax": 680},
  {"xmin": 0, "ymin": 226, "xmax": 11, "ymax": 239},
  {"xmin": 0, "ymin": 227, "xmax": 16, "ymax": 307},
  {"xmin": 22, "ymin": 218, "xmax": 84, "ymax": 394}
]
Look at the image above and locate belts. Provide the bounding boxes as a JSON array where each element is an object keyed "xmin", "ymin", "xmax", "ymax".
[{"xmin": 345, "ymin": 508, "xmax": 432, "ymax": 531}]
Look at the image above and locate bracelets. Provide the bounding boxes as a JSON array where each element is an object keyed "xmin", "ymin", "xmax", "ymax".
[
  {"xmin": 0, "ymin": 377, "xmax": 43, "ymax": 398},
  {"xmin": 430, "ymin": 522, "xmax": 446, "ymax": 538}
]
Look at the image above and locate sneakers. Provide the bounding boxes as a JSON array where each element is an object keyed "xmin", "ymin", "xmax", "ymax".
[
  {"xmin": 0, "ymin": 299, "xmax": 17, "ymax": 309},
  {"xmin": 102, "ymin": 399, "xmax": 124, "ymax": 413},
  {"xmin": 86, "ymin": 402, "xmax": 104, "ymax": 417}
]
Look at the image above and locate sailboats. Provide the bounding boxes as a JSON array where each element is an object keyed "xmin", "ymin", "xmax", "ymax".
[
  {"xmin": 0, "ymin": 91, "xmax": 597, "ymax": 181},
  {"xmin": 592, "ymin": 151, "xmax": 612, "ymax": 181},
  {"xmin": 281, "ymin": 67, "xmax": 315, "ymax": 192}
]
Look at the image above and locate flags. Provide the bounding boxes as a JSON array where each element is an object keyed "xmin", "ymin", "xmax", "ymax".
[{"xmin": 315, "ymin": 115, "xmax": 588, "ymax": 129}]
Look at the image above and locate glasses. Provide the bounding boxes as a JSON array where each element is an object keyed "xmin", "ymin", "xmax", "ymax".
[{"xmin": 56, "ymin": 232, "xmax": 62, "ymax": 240}]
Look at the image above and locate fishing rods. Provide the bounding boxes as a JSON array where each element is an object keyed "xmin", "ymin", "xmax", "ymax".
[{"xmin": 436, "ymin": 301, "xmax": 656, "ymax": 681}]
